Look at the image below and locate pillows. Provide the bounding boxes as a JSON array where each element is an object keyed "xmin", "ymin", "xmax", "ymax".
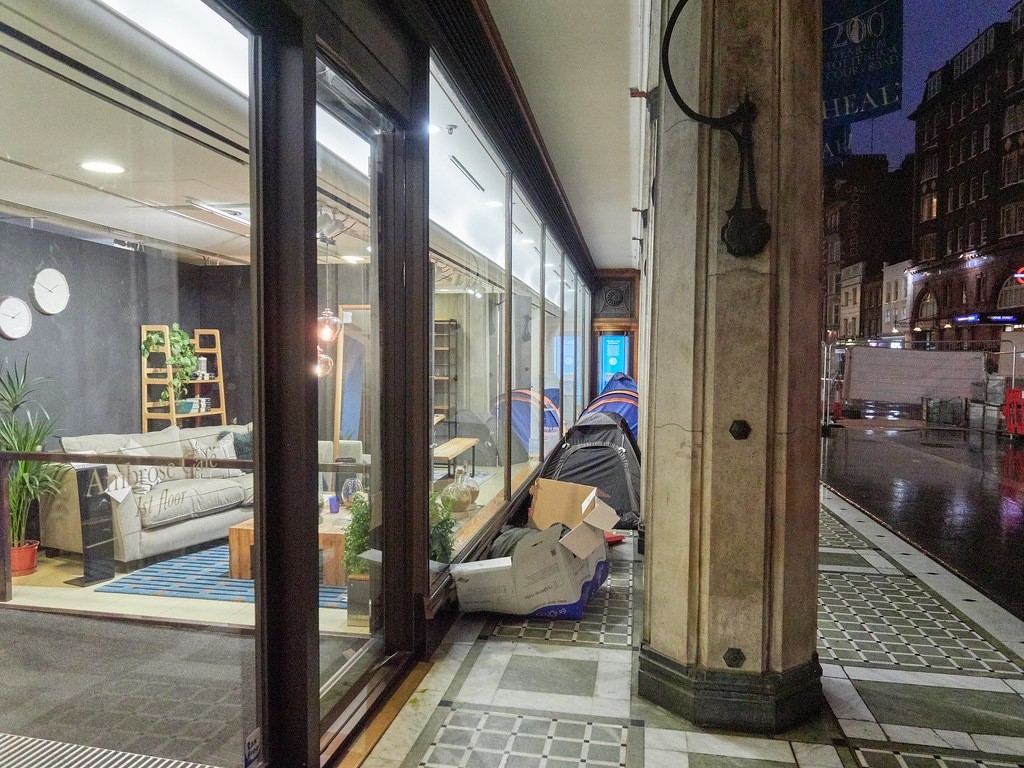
[
  {"xmin": 189, "ymin": 433, "xmax": 245, "ymax": 479},
  {"xmin": 217, "ymin": 430, "xmax": 254, "ymax": 473},
  {"xmin": 115, "ymin": 438, "xmax": 161, "ymax": 496}
]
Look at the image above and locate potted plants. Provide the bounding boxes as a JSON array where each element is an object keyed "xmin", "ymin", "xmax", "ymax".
[
  {"xmin": 140, "ymin": 322, "xmax": 200, "ymax": 407},
  {"xmin": 341, "ymin": 492, "xmax": 372, "ymax": 628},
  {"xmin": 0, "ymin": 352, "xmax": 75, "ymax": 578}
]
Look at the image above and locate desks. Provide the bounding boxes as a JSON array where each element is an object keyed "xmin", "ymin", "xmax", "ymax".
[{"xmin": 434, "ymin": 413, "xmax": 446, "ymax": 426}]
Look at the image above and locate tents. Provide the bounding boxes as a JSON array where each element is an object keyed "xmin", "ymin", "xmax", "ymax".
[
  {"xmin": 601, "ymin": 371, "xmax": 637, "ymax": 394},
  {"xmin": 507, "ymin": 411, "xmax": 641, "ymax": 529},
  {"xmin": 435, "ymin": 409, "xmax": 529, "ymax": 467},
  {"xmin": 490, "ymin": 388, "xmax": 560, "ymax": 453},
  {"xmin": 577, "ymin": 389, "xmax": 639, "ymax": 438}
]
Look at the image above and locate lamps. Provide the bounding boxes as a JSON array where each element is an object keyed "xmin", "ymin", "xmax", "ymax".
[
  {"xmin": 913, "ymin": 322, "xmax": 922, "ymax": 332},
  {"xmin": 430, "ymin": 140, "xmax": 485, "ymax": 197},
  {"xmin": 943, "ymin": 312, "xmax": 953, "ymax": 328},
  {"xmin": 317, "ymin": 345, "xmax": 334, "ymax": 378},
  {"xmin": 451, "ymin": 272, "xmax": 461, "ymax": 287},
  {"xmin": 467, "ymin": 282, "xmax": 486, "ymax": 299},
  {"xmin": 317, "ymin": 201, "xmax": 350, "ymax": 238},
  {"xmin": 317, "ymin": 238, "xmax": 345, "ymax": 341},
  {"xmin": 891, "ymin": 320, "xmax": 899, "ymax": 333},
  {"xmin": 435, "ymin": 265, "xmax": 443, "ymax": 280}
]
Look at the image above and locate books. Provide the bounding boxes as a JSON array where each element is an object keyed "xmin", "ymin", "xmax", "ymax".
[
  {"xmin": 188, "ymin": 397, "xmax": 212, "ymax": 413},
  {"xmin": 197, "ymin": 373, "xmax": 215, "ymax": 380}
]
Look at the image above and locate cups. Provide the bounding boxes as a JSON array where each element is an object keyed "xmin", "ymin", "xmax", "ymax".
[{"xmin": 329, "ymin": 496, "xmax": 340, "ymax": 513}]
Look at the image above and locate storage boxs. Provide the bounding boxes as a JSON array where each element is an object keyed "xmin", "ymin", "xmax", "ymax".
[
  {"xmin": 175, "ymin": 372, "xmax": 216, "ymax": 414},
  {"xmin": 450, "ymin": 476, "xmax": 620, "ymax": 621}
]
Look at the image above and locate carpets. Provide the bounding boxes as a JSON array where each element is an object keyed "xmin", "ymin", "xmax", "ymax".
[
  {"xmin": 433, "ymin": 470, "xmax": 497, "ymax": 485},
  {"xmin": 93, "ymin": 542, "xmax": 347, "ymax": 610}
]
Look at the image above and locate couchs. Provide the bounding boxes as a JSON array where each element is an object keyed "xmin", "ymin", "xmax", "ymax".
[{"xmin": 37, "ymin": 422, "xmax": 333, "ymax": 576}]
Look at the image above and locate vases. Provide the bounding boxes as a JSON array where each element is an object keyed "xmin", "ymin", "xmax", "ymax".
[{"xmin": 340, "ymin": 478, "xmax": 364, "ymax": 509}]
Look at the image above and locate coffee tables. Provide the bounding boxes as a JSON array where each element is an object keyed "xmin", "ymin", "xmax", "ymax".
[{"xmin": 228, "ymin": 491, "xmax": 368, "ymax": 587}]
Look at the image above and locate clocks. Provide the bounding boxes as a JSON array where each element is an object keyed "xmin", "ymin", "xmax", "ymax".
[
  {"xmin": 34, "ymin": 268, "xmax": 69, "ymax": 315},
  {"xmin": 0, "ymin": 298, "xmax": 31, "ymax": 337}
]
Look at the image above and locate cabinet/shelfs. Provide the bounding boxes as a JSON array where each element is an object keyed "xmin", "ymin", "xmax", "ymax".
[
  {"xmin": 141, "ymin": 325, "xmax": 227, "ymax": 434},
  {"xmin": 434, "ymin": 318, "xmax": 458, "ymax": 465}
]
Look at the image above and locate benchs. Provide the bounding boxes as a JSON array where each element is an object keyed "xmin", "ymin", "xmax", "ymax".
[{"xmin": 434, "ymin": 438, "xmax": 480, "ymax": 479}]
